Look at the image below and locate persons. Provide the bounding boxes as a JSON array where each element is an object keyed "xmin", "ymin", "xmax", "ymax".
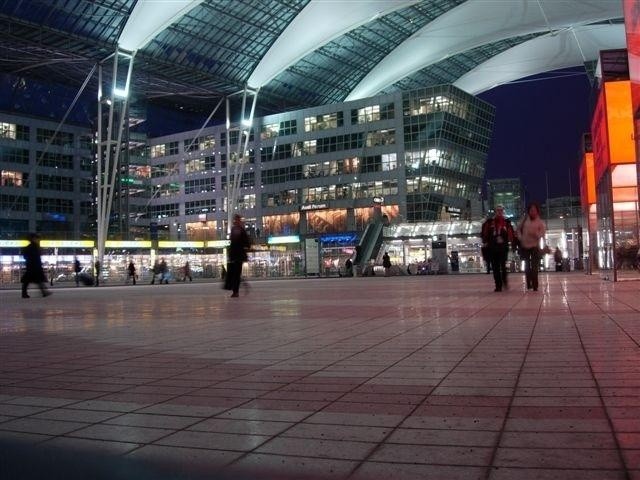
[
  {"xmin": 382, "ymin": 252, "xmax": 391, "ymax": 276},
  {"xmin": 516, "ymin": 202, "xmax": 551, "ymax": 292},
  {"xmin": 480, "ymin": 205, "xmax": 516, "ymax": 292},
  {"xmin": 554, "ymin": 247, "xmax": 562, "ymax": 272},
  {"xmin": 346, "ymin": 259, "xmax": 353, "ymax": 276},
  {"xmin": 221, "ymin": 213, "xmax": 253, "ymax": 299},
  {"xmin": 20, "ymin": 231, "xmax": 53, "ymax": 300},
  {"xmin": 75, "ymin": 258, "xmax": 225, "ymax": 286},
  {"xmin": 382, "ymin": 212, "xmax": 389, "ymax": 227},
  {"xmin": 355, "ymin": 243, "xmax": 363, "ymax": 260}
]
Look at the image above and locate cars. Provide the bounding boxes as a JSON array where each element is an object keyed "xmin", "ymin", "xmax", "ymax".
[{"xmin": 10, "ymin": 260, "xmax": 76, "ymax": 274}]
